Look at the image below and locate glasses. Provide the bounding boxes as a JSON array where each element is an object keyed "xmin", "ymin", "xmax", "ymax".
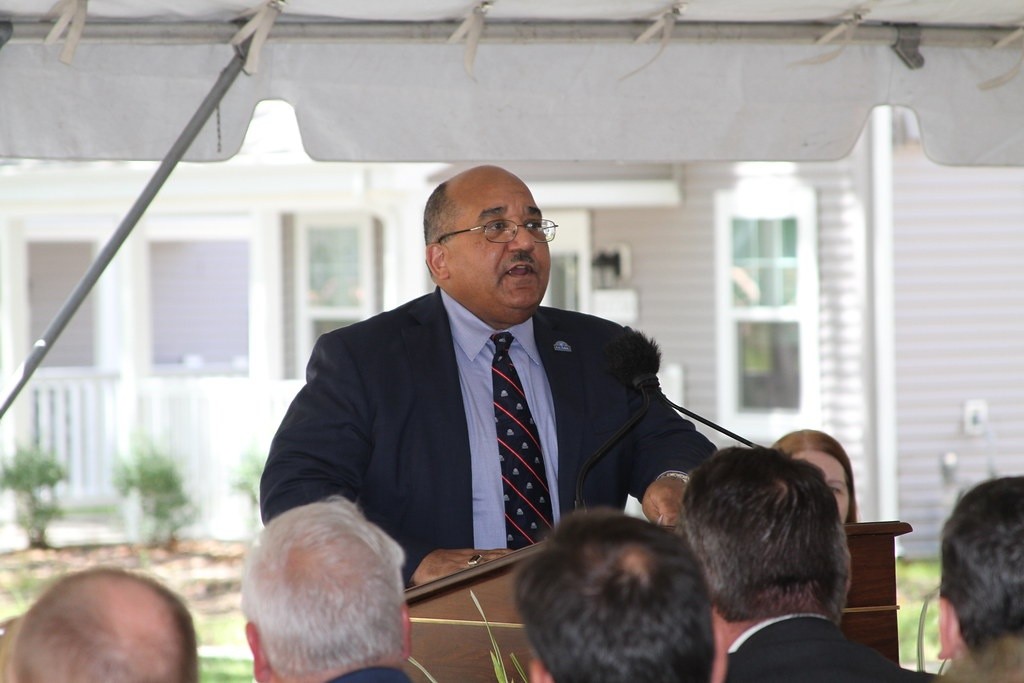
[{"xmin": 437, "ymin": 219, "xmax": 557, "ymax": 244}]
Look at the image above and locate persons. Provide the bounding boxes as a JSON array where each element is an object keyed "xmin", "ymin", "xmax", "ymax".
[
  {"xmin": 512, "ymin": 507, "xmax": 726, "ymax": 683},
  {"xmin": 769, "ymin": 429, "xmax": 857, "ymax": 526},
  {"xmin": 259, "ymin": 165, "xmax": 718, "ymax": 586},
  {"xmin": 242, "ymin": 495, "xmax": 415, "ymax": 683},
  {"xmin": 5, "ymin": 568, "xmax": 198, "ymax": 683},
  {"xmin": 938, "ymin": 475, "xmax": 1024, "ymax": 683},
  {"xmin": 673, "ymin": 446, "xmax": 944, "ymax": 683}
]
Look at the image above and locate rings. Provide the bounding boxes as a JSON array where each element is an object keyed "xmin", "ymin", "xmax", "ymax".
[{"xmin": 468, "ymin": 555, "xmax": 483, "ymax": 567}]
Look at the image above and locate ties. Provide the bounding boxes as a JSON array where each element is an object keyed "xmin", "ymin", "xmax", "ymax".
[{"xmin": 489, "ymin": 330, "xmax": 553, "ymax": 552}]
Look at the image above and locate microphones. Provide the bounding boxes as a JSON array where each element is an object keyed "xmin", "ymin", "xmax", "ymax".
[{"xmin": 574, "ymin": 331, "xmax": 760, "ymax": 521}]
